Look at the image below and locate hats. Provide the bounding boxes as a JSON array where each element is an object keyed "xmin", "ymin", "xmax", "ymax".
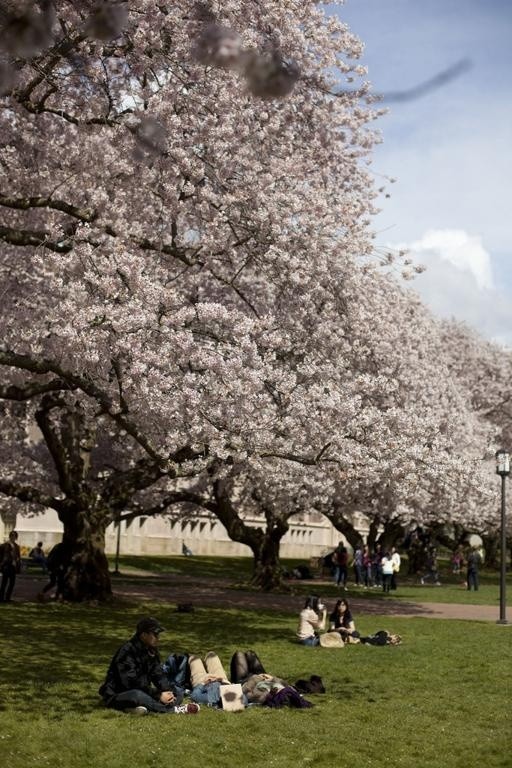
[{"xmin": 136, "ymin": 618, "xmax": 164, "ymax": 633}]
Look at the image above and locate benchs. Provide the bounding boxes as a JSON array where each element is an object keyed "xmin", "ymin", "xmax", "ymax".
[{"xmin": 20, "ymin": 558, "xmax": 47, "ymax": 571}]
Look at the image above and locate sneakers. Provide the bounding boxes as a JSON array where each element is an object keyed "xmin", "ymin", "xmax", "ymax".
[
  {"xmin": 334, "ymin": 582, "xmax": 382, "ymax": 591},
  {"xmin": 125, "ymin": 705, "xmax": 148, "ymax": 714},
  {"xmin": 174, "ymin": 703, "xmax": 200, "ymax": 714}
]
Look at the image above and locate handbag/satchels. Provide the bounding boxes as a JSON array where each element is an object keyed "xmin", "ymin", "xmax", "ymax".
[{"xmin": 319, "ymin": 632, "xmax": 345, "ymax": 647}]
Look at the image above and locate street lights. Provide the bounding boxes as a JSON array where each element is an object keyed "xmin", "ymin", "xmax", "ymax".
[{"xmin": 494, "ymin": 447, "xmax": 510, "ymax": 626}]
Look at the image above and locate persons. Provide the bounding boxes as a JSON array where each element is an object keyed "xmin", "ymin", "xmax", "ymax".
[
  {"xmin": 37, "ymin": 536, "xmax": 72, "ymax": 604},
  {"xmin": 98, "ymin": 618, "xmax": 314, "ymax": 717},
  {"xmin": 296, "ymin": 595, "xmax": 402, "ymax": 647},
  {"xmin": 323, "ymin": 541, "xmax": 480, "ymax": 591},
  {"xmin": 0, "ymin": 531, "xmax": 44, "ymax": 602}
]
